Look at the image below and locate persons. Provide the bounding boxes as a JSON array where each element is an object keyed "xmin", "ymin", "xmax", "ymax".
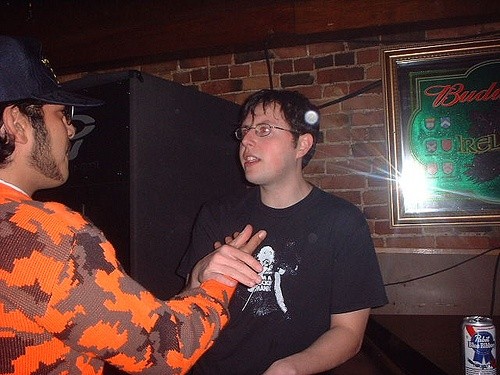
[
  {"xmin": 175, "ymin": 90, "xmax": 390, "ymax": 375},
  {"xmin": 0, "ymin": 36, "xmax": 268, "ymax": 375}
]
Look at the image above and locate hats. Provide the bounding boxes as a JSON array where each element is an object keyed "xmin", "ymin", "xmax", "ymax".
[{"xmin": 1, "ymin": 36, "xmax": 107, "ymax": 106}]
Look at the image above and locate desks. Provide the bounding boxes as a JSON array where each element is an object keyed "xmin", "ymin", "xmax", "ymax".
[{"xmin": 364, "ymin": 313, "xmax": 500, "ymax": 375}]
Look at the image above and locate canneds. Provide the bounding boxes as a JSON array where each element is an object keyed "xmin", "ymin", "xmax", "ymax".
[{"xmin": 461, "ymin": 315, "xmax": 497, "ymax": 375}]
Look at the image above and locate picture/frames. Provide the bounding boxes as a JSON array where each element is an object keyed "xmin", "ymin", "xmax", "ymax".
[{"xmin": 377, "ymin": 35, "xmax": 500, "ymax": 230}]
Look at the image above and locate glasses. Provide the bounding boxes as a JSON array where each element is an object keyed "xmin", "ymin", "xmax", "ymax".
[{"xmin": 235, "ymin": 123, "xmax": 305, "ymax": 139}]
